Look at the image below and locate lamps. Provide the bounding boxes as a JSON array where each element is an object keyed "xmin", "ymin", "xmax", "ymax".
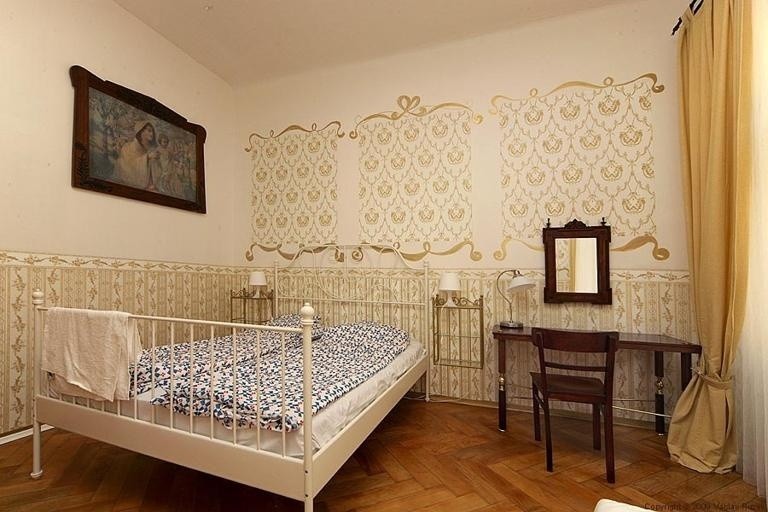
[
  {"xmin": 438, "ymin": 271, "xmax": 464, "ymax": 308},
  {"xmin": 497, "ymin": 270, "xmax": 537, "ymax": 328},
  {"xmin": 247, "ymin": 271, "xmax": 269, "ymax": 299}
]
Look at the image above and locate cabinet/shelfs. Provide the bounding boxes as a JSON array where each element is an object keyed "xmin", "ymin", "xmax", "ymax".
[
  {"xmin": 433, "ymin": 294, "xmax": 485, "ymax": 370},
  {"xmin": 228, "ymin": 287, "xmax": 275, "ymax": 334}
]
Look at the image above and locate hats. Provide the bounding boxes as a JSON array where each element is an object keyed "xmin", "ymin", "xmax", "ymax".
[{"xmin": 133, "ymin": 120, "xmax": 156, "ymax": 138}]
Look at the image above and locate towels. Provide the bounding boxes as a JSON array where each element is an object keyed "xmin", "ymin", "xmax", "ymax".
[{"xmin": 38, "ymin": 303, "xmax": 141, "ymax": 403}]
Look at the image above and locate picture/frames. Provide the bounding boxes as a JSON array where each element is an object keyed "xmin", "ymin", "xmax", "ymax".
[{"xmin": 69, "ymin": 65, "xmax": 210, "ymax": 216}]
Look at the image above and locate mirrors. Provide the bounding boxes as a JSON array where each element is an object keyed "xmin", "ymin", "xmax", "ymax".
[{"xmin": 541, "ymin": 216, "xmax": 614, "ymax": 306}]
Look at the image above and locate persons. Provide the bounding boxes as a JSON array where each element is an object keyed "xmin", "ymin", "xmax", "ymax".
[{"xmin": 113, "ymin": 121, "xmax": 183, "ymax": 197}]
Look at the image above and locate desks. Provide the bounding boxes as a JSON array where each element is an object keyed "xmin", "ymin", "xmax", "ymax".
[{"xmin": 491, "ymin": 323, "xmax": 704, "ymax": 434}]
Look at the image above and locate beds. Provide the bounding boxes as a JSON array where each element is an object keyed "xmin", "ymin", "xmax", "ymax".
[{"xmin": 26, "ymin": 242, "xmax": 433, "ymax": 511}]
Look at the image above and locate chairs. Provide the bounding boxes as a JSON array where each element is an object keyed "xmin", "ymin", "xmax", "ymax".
[{"xmin": 527, "ymin": 326, "xmax": 621, "ymax": 483}]
